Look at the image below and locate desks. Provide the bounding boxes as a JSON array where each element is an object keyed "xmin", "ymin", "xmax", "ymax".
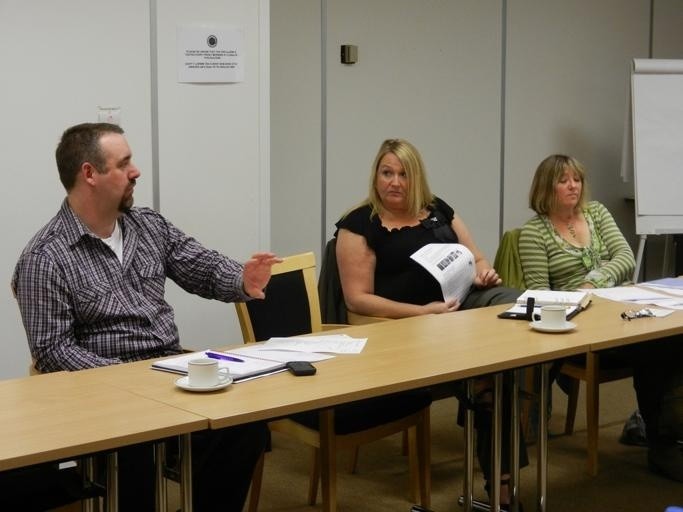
[
  {"xmin": 84, "ymin": 308, "xmax": 590, "ymax": 510},
  {"xmin": 1, "ymin": 369, "xmax": 209, "ymax": 511},
  {"xmin": 471, "ymin": 275, "xmax": 683, "ymax": 511}
]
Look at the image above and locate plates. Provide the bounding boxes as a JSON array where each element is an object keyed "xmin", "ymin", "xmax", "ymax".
[
  {"xmin": 173, "ymin": 376, "xmax": 233, "ymax": 392},
  {"xmin": 529, "ymin": 322, "xmax": 576, "ymax": 332}
]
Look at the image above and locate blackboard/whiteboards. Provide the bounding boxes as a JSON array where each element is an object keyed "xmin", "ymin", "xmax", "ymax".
[{"xmin": 629, "ymin": 54, "xmax": 683, "ymax": 235}]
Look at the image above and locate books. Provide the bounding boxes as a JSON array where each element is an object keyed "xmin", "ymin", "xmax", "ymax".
[
  {"xmin": 498, "ymin": 286, "xmax": 594, "ymax": 322},
  {"xmin": 149, "ymin": 342, "xmax": 337, "ymax": 384}
]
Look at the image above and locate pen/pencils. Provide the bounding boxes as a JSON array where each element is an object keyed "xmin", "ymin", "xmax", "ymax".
[
  {"xmin": 521, "ymin": 305, "xmax": 542, "ymax": 307},
  {"xmin": 205, "ymin": 351, "xmax": 244, "ymax": 362}
]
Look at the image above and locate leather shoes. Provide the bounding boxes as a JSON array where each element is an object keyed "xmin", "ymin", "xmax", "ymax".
[
  {"xmin": 476, "ymin": 389, "xmax": 493, "ymax": 415},
  {"xmin": 483, "ymin": 481, "xmax": 521, "ymax": 512}
]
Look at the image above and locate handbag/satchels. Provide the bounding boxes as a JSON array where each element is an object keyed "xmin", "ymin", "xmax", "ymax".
[{"xmin": 621, "ymin": 409, "xmax": 646, "ymax": 445}]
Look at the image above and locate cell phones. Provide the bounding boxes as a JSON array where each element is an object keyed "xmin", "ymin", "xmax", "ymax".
[{"xmin": 286, "ymin": 361, "xmax": 316, "ymax": 376}]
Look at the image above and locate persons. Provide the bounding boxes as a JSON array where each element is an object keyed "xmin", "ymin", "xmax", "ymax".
[
  {"xmin": 518, "ymin": 153, "xmax": 682, "ymax": 485},
  {"xmin": 334, "ymin": 139, "xmax": 529, "ymax": 511},
  {"xmin": 10, "ymin": 124, "xmax": 283, "ymax": 512}
]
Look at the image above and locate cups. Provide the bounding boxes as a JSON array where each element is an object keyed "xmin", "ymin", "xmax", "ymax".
[
  {"xmin": 188, "ymin": 359, "xmax": 229, "ymax": 386},
  {"xmin": 532, "ymin": 305, "xmax": 566, "ymax": 327}
]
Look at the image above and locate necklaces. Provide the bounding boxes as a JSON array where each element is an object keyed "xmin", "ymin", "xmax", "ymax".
[{"xmin": 566, "ymin": 219, "xmax": 578, "ymax": 240}]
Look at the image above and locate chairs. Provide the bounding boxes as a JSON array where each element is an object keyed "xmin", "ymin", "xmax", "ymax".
[{"xmin": 233, "ymin": 249, "xmax": 434, "ymax": 512}]
